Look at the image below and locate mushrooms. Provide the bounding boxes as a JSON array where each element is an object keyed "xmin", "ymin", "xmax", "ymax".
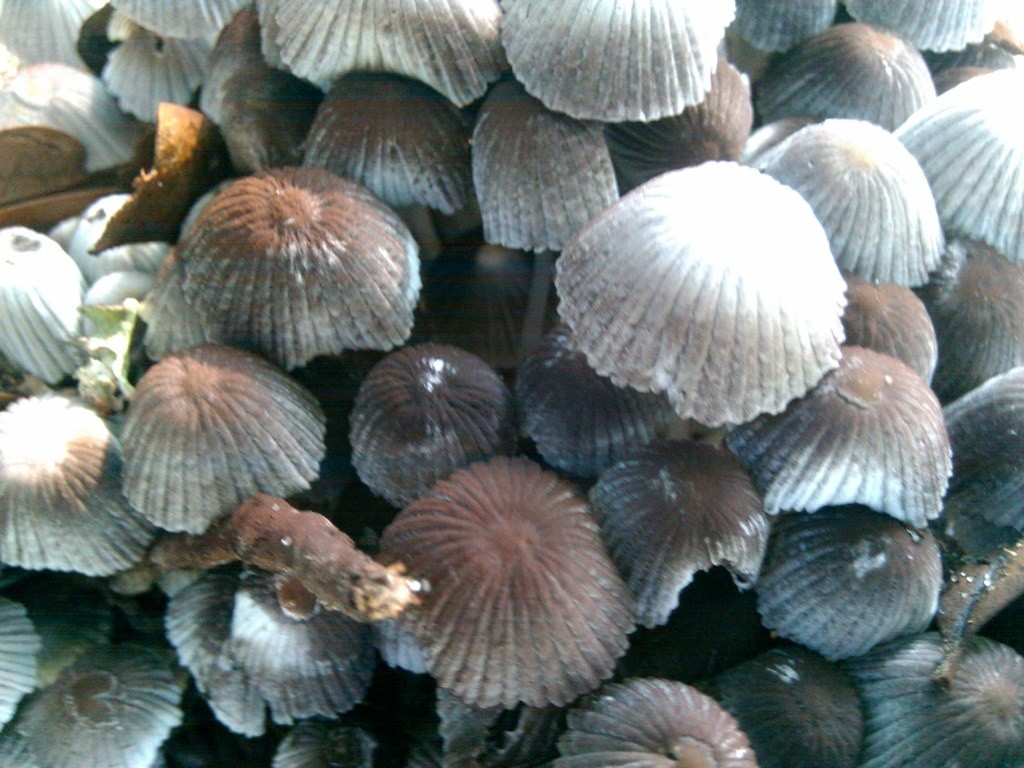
[{"xmin": 0, "ymin": 0, "xmax": 1024, "ymax": 768}]
